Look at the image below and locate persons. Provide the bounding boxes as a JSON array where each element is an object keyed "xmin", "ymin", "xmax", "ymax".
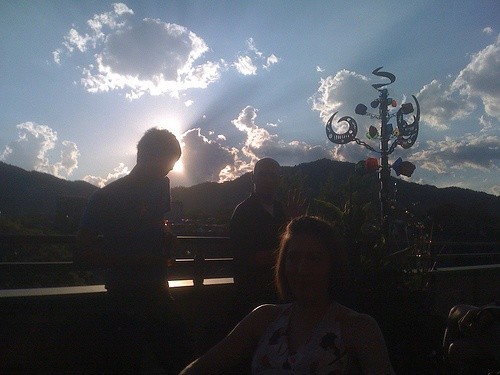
[
  {"xmin": 178, "ymin": 215, "xmax": 401, "ymax": 375},
  {"xmin": 75, "ymin": 127, "xmax": 184, "ymax": 375},
  {"xmin": 232, "ymin": 157, "xmax": 311, "ymax": 314}
]
